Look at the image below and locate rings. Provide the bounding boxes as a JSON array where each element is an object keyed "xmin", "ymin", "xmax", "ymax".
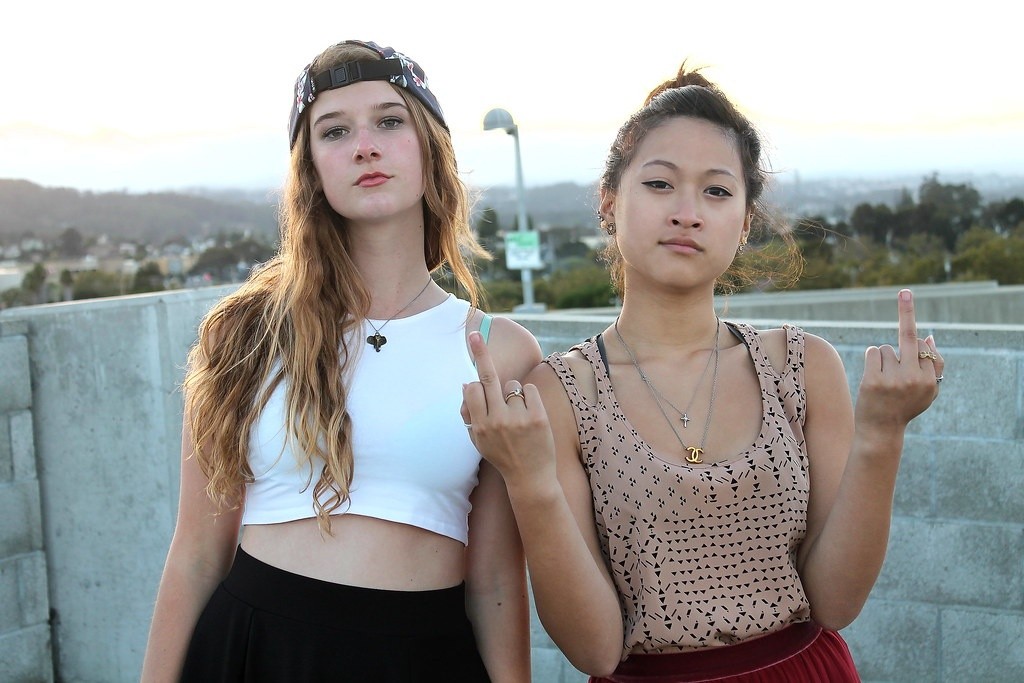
[
  {"xmin": 919, "ymin": 350, "xmax": 937, "ymax": 362},
  {"xmin": 504, "ymin": 388, "xmax": 525, "ymax": 403},
  {"xmin": 936, "ymin": 376, "xmax": 944, "ymax": 384},
  {"xmin": 464, "ymin": 421, "xmax": 472, "ymax": 430}
]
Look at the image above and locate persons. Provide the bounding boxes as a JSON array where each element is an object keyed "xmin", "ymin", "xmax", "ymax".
[
  {"xmin": 461, "ymin": 70, "xmax": 945, "ymax": 683},
  {"xmin": 140, "ymin": 42, "xmax": 544, "ymax": 683}
]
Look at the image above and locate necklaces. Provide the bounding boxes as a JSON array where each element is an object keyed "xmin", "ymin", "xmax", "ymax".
[
  {"xmin": 613, "ymin": 315, "xmax": 719, "ymax": 465},
  {"xmin": 363, "ymin": 275, "xmax": 433, "ymax": 352}
]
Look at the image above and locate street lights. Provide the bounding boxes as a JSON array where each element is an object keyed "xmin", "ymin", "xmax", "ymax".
[{"xmin": 482, "ymin": 108, "xmax": 548, "ymax": 319}]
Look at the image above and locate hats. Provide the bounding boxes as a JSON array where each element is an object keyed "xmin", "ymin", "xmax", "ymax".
[{"xmin": 289, "ymin": 39, "xmax": 451, "ymax": 155}]
